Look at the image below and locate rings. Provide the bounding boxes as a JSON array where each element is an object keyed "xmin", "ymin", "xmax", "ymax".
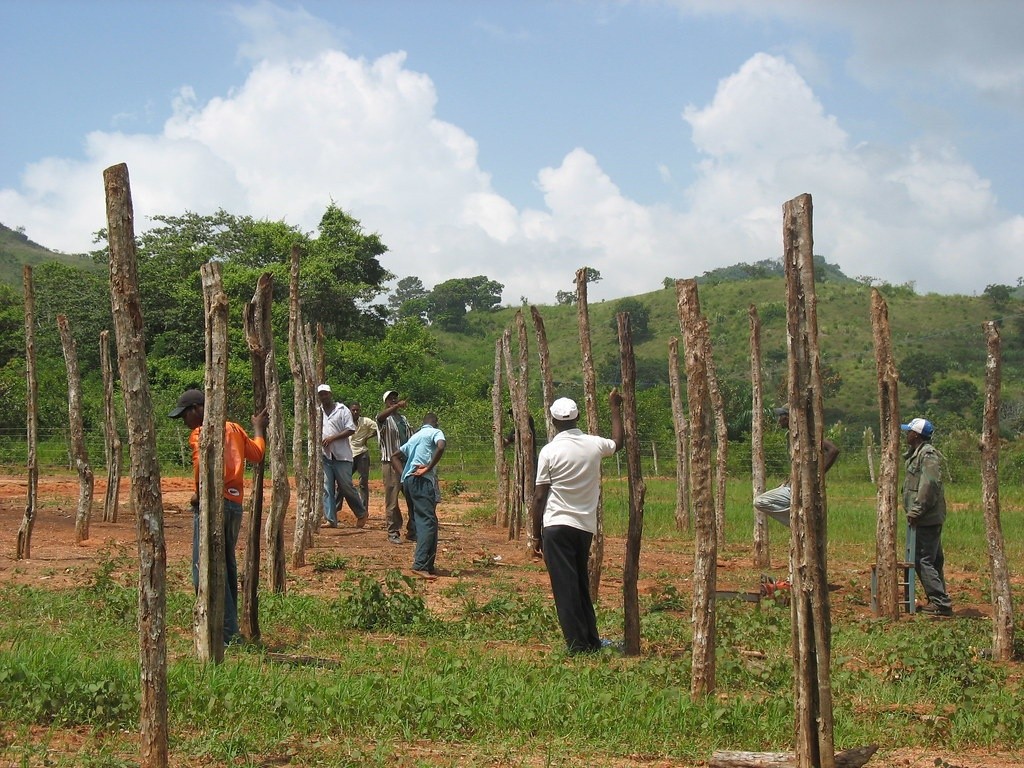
[{"xmin": 325, "ymin": 441, "xmax": 326, "ymax": 442}]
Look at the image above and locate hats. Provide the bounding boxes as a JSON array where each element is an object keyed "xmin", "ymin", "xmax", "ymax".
[
  {"xmin": 549, "ymin": 396, "xmax": 579, "ymax": 422},
  {"xmin": 382, "ymin": 390, "xmax": 399, "ymax": 402},
  {"xmin": 167, "ymin": 388, "xmax": 205, "ymax": 418},
  {"xmin": 773, "ymin": 402, "xmax": 791, "ymax": 416},
  {"xmin": 317, "ymin": 384, "xmax": 331, "ymax": 393},
  {"xmin": 900, "ymin": 417, "xmax": 934, "ymax": 438}
]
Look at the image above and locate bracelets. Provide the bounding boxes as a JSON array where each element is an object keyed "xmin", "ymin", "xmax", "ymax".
[{"xmin": 532, "ymin": 538, "xmax": 540, "ymax": 541}]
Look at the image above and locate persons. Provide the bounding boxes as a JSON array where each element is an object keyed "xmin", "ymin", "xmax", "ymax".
[
  {"xmin": 375, "ymin": 390, "xmax": 417, "ymax": 544},
  {"xmin": 754, "ymin": 402, "xmax": 840, "ymax": 589},
  {"xmin": 316, "ymin": 384, "xmax": 369, "ymax": 529},
  {"xmin": 901, "ymin": 418, "xmax": 955, "ymax": 616},
  {"xmin": 322, "ymin": 401, "xmax": 378, "ymax": 523},
  {"xmin": 390, "ymin": 414, "xmax": 446, "ymax": 580},
  {"xmin": 531, "ymin": 386, "xmax": 622, "ymax": 660},
  {"xmin": 492, "ymin": 409, "xmax": 538, "ymax": 507},
  {"xmin": 168, "ymin": 389, "xmax": 269, "ymax": 646}
]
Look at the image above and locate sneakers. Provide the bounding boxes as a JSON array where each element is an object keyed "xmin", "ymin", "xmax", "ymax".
[{"xmin": 920, "ymin": 600, "xmax": 956, "ymax": 616}]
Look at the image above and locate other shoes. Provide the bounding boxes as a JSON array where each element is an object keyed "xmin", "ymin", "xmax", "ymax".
[
  {"xmin": 388, "ymin": 535, "xmax": 403, "ymax": 544},
  {"xmin": 320, "ymin": 520, "xmax": 338, "ymax": 530},
  {"xmin": 356, "ymin": 512, "xmax": 370, "ymax": 529},
  {"xmin": 410, "ymin": 568, "xmax": 438, "ymax": 582},
  {"xmin": 406, "ymin": 533, "xmax": 418, "ymax": 543}
]
